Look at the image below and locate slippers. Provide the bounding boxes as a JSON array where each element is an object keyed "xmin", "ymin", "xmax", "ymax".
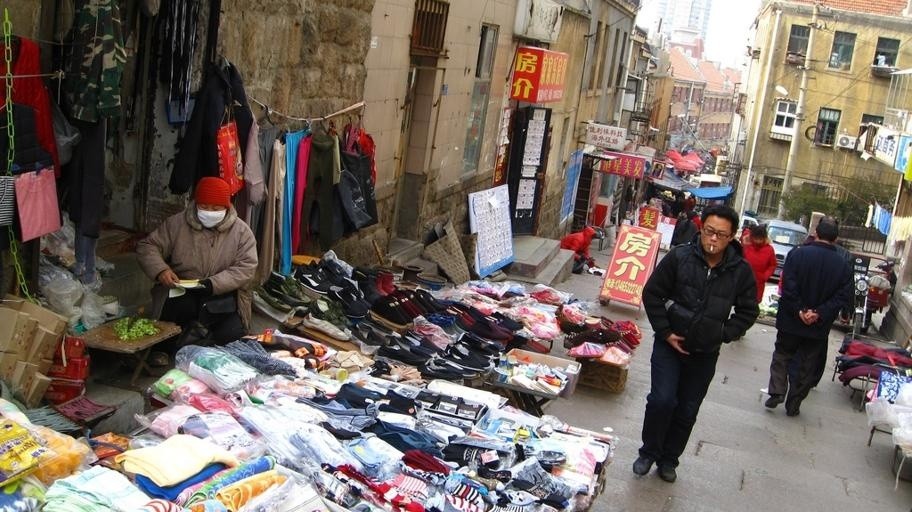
[{"xmin": 563, "ymin": 314, "xmax": 643, "ymax": 366}]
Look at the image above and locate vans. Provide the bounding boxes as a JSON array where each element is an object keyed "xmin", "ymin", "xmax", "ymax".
[{"xmin": 760, "ymin": 216, "xmax": 809, "ymax": 280}]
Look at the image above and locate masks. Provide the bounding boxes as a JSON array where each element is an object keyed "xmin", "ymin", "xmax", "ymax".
[{"xmin": 196, "ymin": 207, "xmax": 227, "ymax": 229}]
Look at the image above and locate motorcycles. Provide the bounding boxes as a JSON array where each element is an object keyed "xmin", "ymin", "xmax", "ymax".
[{"xmin": 836, "ymin": 247, "xmax": 898, "ymax": 342}]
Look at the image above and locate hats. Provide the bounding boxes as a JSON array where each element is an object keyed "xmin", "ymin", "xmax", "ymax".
[{"xmin": 193, "ymin": 175, "xmax": 233, "ymax": 207}]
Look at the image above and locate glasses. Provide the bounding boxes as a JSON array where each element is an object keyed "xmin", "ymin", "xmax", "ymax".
[{"xmin": 703, "ymin": 226, "xmax": 733, "ymax": 240}]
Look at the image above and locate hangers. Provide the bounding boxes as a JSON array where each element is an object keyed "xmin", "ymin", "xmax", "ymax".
[
  {"xmin": 255, "ymin": 103, "xmax": 365, "ymax": 139},
  {"xmin": 2, "ymin": 31, "xmax": 74, "ymax": 82}
]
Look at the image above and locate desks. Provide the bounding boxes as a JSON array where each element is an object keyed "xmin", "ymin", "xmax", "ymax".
[
  {"xmin": 72, "ymin": 315, "xmax": 182, "ymax": 387},
  {"xmin": 485, "ymin": 354, "xmax": 568, "ymax": 419},
  {"xmin": 892, "ymin": 438, "xmax": 912, "ymax": 495},
  {"xmin": 864, "ymin": 409, "xmax": 896, "ymax": 447},
  {"xmin": 281, "ymin": 311, "xmax": 306, "ymax": 331},
  {"xmin": 300, "ymin": 321, "xmax": 372, "ymax": 357}
]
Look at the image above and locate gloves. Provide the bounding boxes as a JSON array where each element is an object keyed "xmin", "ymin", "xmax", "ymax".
[{"xmin": 188, "ymin": 278, "xmax": 213, "ymax": 297}]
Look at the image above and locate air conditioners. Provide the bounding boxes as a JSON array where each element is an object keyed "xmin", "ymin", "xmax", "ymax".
[{"xmin": 836, "ymin": 132, "xmax": 859, "ymax": 152}]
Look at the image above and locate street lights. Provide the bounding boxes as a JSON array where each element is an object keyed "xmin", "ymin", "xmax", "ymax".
[{"xmin": 774, "ymin": 80, "xmax": 810, "ymax": 221}]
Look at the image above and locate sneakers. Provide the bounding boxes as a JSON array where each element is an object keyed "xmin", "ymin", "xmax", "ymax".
[
  {"xmin": 148, "ymin": 351, "xmax": 170, "ymax": 367},
  {"xmin": 257, "ymin": 257, "xmax": 526, "ymax": 383}
]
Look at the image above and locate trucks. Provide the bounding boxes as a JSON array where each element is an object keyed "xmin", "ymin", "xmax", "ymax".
[{"xmin": 736, "ymin": 210, "xmax": 764, "ymax": 245}]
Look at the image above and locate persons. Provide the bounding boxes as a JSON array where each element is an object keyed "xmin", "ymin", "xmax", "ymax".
[
  {"xmin": 633, "ymin": 205, "xmax": 760, "ymax": 483},
  {"xmin": 136, "ymin": 177, "xmax": 258, "ymax": 365},
  {"xmin": 765, "ymin": 219, "xmax": 852, "ymax": 416},
  {"xmin": 803, "ymin": 215, "xmax": 854, "ymax": 388},
  {"xmin": 658, "ymin": 190, "xmax": 778, "ymax": 301},
  {"xmin": 560, "ymin": 226, "xmax": 596, "ymax": 274}
]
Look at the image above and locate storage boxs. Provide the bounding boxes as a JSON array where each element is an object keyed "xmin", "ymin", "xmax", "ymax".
[
  {"xmin": 503, "ymin": 349, "xmax": 583, "ymax": 401},
  {"xmin": 574, "ymin": 357, "xmax": 631, "ymax": 395},
  {"xmin": 0, "ymin": 291, "xmax": 90, "ymax": 406}
]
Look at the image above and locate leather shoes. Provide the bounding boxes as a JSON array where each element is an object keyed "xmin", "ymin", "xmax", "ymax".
[
  {"xmin": 764, "ymin": 394, "xmax": 786, "ymax": 409},
  {"xmin": 786, "ymin": 407, "xmax": 799, "ymax": 416},
  {"xmin": 656, "ymin": 463, "xmax": 678, "ymax": 482},
  {"xmin": 632, "ymin": 455, "xmax": 654, "ymax": 475}
]
